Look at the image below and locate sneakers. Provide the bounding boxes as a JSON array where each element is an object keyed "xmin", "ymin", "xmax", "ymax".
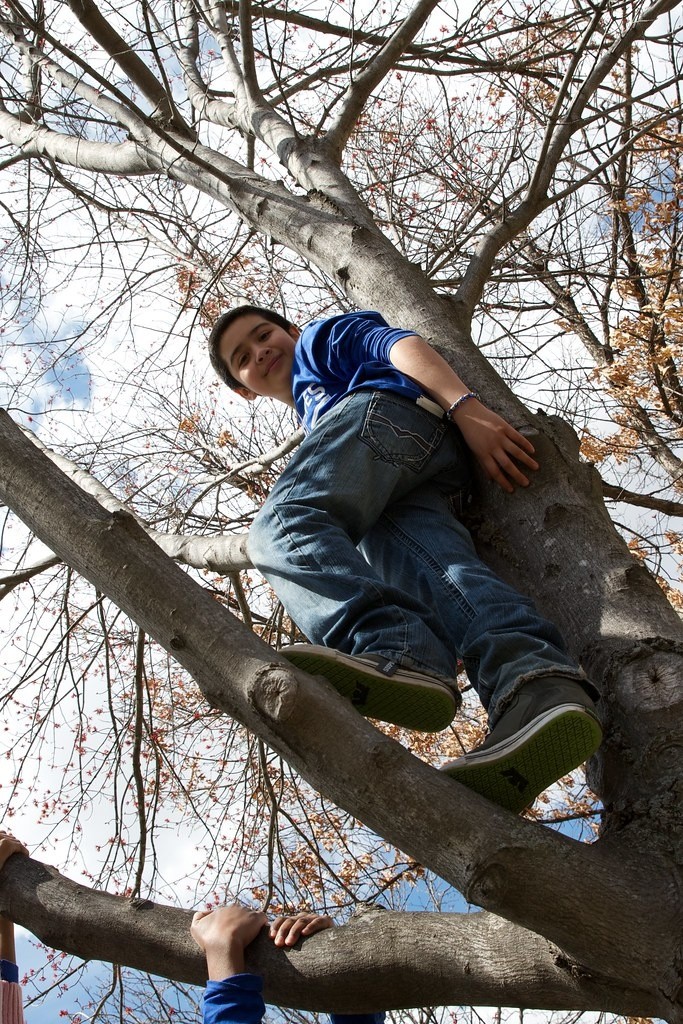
[
  {"xmin": 438, "ymin": 676, "xmax": 605, "ymax": 814},
  {"xmin": 276, "ymin": 644, "xmax": 459, "ymax": 732}
]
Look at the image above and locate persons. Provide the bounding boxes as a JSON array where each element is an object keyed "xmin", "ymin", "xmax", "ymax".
[
  {"xmin": 190, "ymin": 903, "xmax": 386, "ymax": 1024},
  {"xmin": 0, "ymin": 831, "xmax": 29, "ymax": 1023},
  {"xmin": 208, "ymin": 306, "xmax": 605, "ymax": 815}
]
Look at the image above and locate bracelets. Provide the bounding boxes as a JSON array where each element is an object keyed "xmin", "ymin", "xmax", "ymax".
[{"xmin": 446, "ymin": 391, "xmax": 481, "ymax": 422}]
